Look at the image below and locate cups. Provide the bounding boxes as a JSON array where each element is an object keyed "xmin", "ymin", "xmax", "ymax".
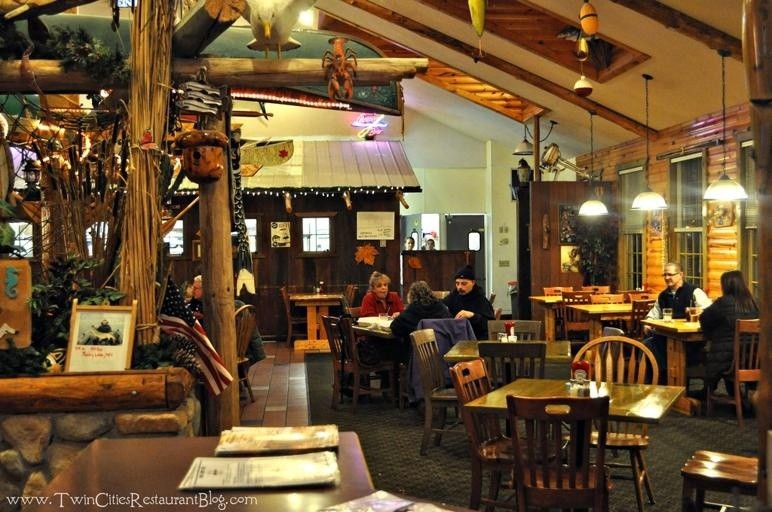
[
  {"xmin": 685, "ymin": 307, "xmax": 696, "ymax": 321},
  {"xmin": 496, "ymin": 332, "xmax": 504, "ymax": 343},
  {"xmin": 690, "ymin": 315, "xmax": 698, "ymax": 322},
  {"xmin": 662, "ymin": 308, "xmax": 672, "ymax": 323},
  {"xmin": 378, "ymin": 313, "xmax": 388, "ymax": 323}
]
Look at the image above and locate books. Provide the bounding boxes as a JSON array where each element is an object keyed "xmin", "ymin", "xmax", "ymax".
[
  {"xmin": 214, "ymin": 421, "xmax": 341, "ymax": 454},
  {"xmin": 175, "ymin": 451, "xmax": 339, "ymax": 491},
  {"xmin": 319, "ymin": 490, "xmax": 420, "ymax": 512}
]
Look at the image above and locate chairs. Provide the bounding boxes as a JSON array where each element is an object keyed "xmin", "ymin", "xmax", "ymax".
[
  {"xmin": 279, "ymin": 284, "xmax": 761, "ymax": 512},
  {"xmin": 235, "ymin": 304, "xmax": 257, "ymax": 403}
]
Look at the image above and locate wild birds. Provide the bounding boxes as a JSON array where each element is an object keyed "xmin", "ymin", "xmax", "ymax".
[{"xmin": 241, "ymin": 0, "xmax": 316, "ymax": 58}]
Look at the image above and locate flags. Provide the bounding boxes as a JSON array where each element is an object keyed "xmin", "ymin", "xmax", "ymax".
[{"xmin": 158, "ymin": 277, "xmax": 232, "ymax": 397}]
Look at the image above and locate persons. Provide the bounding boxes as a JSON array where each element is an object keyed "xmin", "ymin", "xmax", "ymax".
[
  {"xmin": 233, "ymin": 299, "xmax": 266, "ymax": 401},
  {"xmin": 404, "ymin": 237, "xmax": 414, "ymax": 251},
  {"xmin": 635, "ymin": 261, "xmax": 713, "ymax": 385},
  {"xmin": 439, "ymin": 265, "xmax": 496, "ymax": 340},
  {"xmin": 189, "ymin": 273, "xmax": 204, "ymax": 319},
  {"xmin": 696, "ymin": 268, "xmax": 761, "ymax": 418},
  {"xmin": 357, "ymin": 270, "xmax": 407, "ymax": 399},
  {"xmin": 425, "ymin": 238, "xmax": 435, "ymax": 251},
  {"xmin": 390, "ymin": 279, "xmax": 453, "ymax": 368}
]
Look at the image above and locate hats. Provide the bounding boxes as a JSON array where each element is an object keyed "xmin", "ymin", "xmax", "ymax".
[{"xmin": 454, "ymin": 265, "xmax": 475, "ymax": 280}]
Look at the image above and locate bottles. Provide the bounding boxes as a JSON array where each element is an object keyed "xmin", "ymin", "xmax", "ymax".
[
  {"xmin": 564, "ymin": 378, "xmax": 608, "ymax": 398},
  {"xmin": 315, "ymin": 281, "xmax": 325, "ymax": 294},
  {"xmin": 508, "ymin": 326, "xmax": 518, "ymax": 343},
  {"xmin": 501, "ymin": 334, "xmax": 507, "ymax": 343}
]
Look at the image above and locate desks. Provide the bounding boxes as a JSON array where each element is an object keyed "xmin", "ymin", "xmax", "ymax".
[{"xmin": 22, "ymin": 431, "xmax": 375, "ymax": 512}]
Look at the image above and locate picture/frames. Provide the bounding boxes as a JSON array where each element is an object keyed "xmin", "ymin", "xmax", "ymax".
[{"xmin": 64, "ymin": 298, "xmax": 138, "ymax": 375}]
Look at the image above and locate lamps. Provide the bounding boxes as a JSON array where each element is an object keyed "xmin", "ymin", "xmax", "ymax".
[
  {"xmin": 630, "ymin": 73, "xmax": 667, "ymax": 210},
  {"xmin": 578, "ymin": 110, "xmax": 608, "ymax": 217},
  {"xmin": 513, "ymin": 119, "xmax": 558, "ymax": 156},
  {"xmin": 702, "ymin": 48, "xmax": 748, "ymax": 201}
]
